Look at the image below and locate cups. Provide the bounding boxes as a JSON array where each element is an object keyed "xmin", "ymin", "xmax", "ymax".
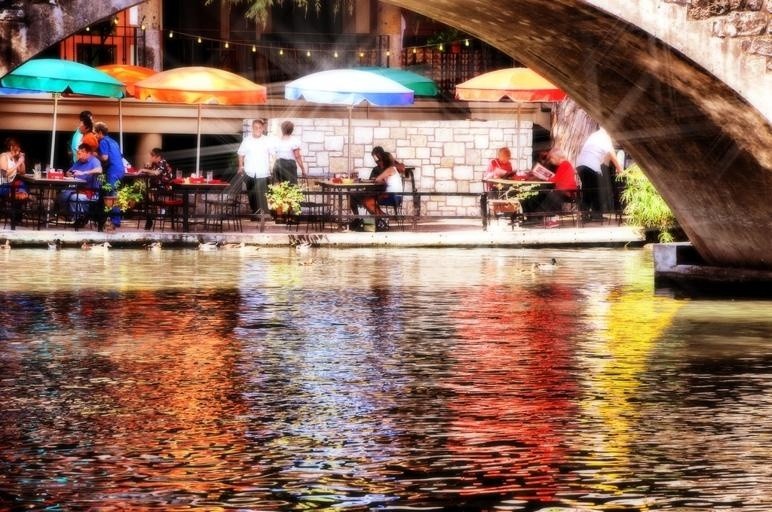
[
  {"xmin": 353, "ymin": 172, "xmax": 359, "ymax": 183},
  {"xmin": 50, "ymin": 168, "xmax": 63, "ymax": 174},
  {"xmin": 34, "ymin": 163, "xmax": 42, "ymax": 179},
  {"xmin": 190, "ymin": 170, "xmax": 214, "ymax": 183},
  {"xmin": 176, "ymin": 170, "xmax": 182, "ymax": 179}
]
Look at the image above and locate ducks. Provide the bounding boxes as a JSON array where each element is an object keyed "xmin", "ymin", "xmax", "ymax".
[
  {"xmin": 537, "ymin": 258, "xmax": 558, "ymax": 271},
  {"xmin": 0, "ymin": 239, "xmax": 12, "ymax": 250},
  {"xmin": 143, "ymin": 241, "xmax": 162, "ymax": 251},
  {"xmin": 92, "ymin": 242, "xmax": 112, "ymax": 251},
  {"xmin": 48, "ymin": 239, "xmax": 64, "ymax": 248},
  {"xmin": 198, "ymin": 240, "xmax": 260, "ymax": 251},
  {"xmin": 81, "ymin": 239, "xmax": 102, "ymax": 248},
  {"xmin": 289, "ymin": 240, "xmax": 313, "ymax": 248}
]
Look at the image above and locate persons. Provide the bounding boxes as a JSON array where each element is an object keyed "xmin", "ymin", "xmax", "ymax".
[
  {"xmin": 0, "ymin": 140, "xmax": 28, "ymax": 225},
  {"xmin": 480, "ymin": 129, "xmax": 620, "ymax": 228},
  {"xmin": 139, "ymin": 149, "xmax": 174, "ymax": 229},
  {"xmin": 349, "ymin": 147, "xmax": 405, "ymax": 231},
  {"xmin": 57, "ymin": 111, "xmax": 132, "ymax": 231},
  {"xmin": 361, "ymin": 152, "xmax": 403, "ymax": 231},
  {"xmin": 236, "ymin": 119, "xmax": 274, "ymax": 221},
  {"xmin": 271, "ymin": 121, "xmax": 308, "ymax": 224}
]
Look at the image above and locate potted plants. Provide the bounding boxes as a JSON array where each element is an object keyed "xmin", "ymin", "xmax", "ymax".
[{"xmin": 616, "ymin": 161, "xmax": 688, "ymax": 243}]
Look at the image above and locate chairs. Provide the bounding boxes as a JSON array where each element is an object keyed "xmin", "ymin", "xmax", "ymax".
[{"xmin": 0, "ymin": 163, "xmax": 628, "ymax": 232}]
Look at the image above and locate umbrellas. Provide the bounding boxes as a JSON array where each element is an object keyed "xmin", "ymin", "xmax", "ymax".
[
  {"xmin": 135, "ymin": 65, "xmax": 268, "ymax": 231},
  {"xmin": 0, "ymin": 59, "xmax": 128, "ymax": 228},
  {"xmin": 366, "ymin": 70, "xmax": 437, "ymax": 96},
  {"xmin": 97, "ymin": 63, "xmax": 159, "ymax": 156},
  {"xmin": 285, "ymin": 68, "xmax": 415, "ymax": 229},
  {"xmin": 454, "ymin": 67, "xmax": 567, "ymax": 167}
]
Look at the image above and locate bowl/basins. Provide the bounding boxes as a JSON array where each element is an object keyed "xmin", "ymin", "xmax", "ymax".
[{"xmin": 342, "ymin": 179, "xmax": 353, "ymax": 184}]
